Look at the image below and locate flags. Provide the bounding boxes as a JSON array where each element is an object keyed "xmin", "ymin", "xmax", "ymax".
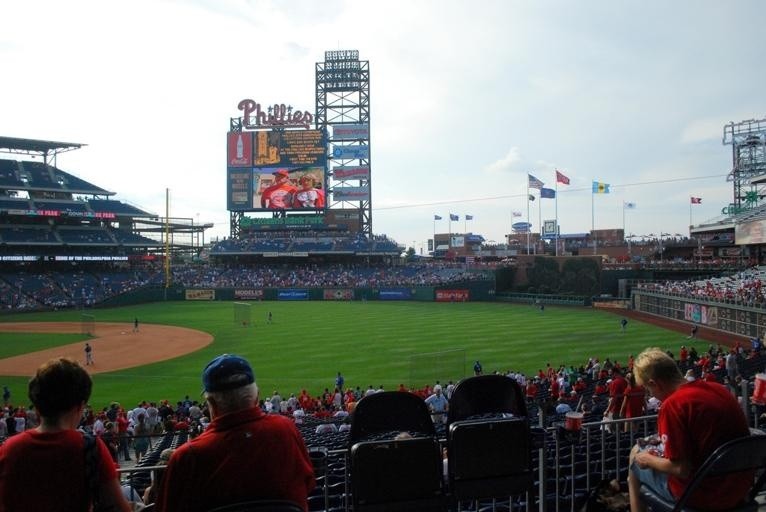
[
  {"xmin": 556, "ymin": 170, "xmax": 570, "ymax": 185},
  {"xmin": 511, "ymin": 210, "xmax": 523, "ymax": 218},
  {"xmin": 449, "ymin": 213, "xmax": 459, "ymax": 221},
  {"xmin": 465, "ymin": 215, "xmax": 474, "ymax": 220},
  {"xmin": 434, "ymin": 214, "xmax": 442, "ymax": 220},
  {"xmin": 529, "ymin": 194, "xmax": 535, "ymax": 201},
  {"xmin": 691, "ymin": 196, "xmax": 702, "ymax": 204},
  {"xmin": 625, "ymin": 201, "xmax": 637, "ymax": 209},
  {"xmin": 528, "ymin": 173, "xmax": 544, "ymax": 190},
  {"xmin": 592, "ymin": 181, "xmax": 611, "ymax": 194},
  {"xmin": 540, "ymin": 187, "xmax": 556, "ymax": 199}
]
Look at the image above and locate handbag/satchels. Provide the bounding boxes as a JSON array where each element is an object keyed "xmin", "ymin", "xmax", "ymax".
[{"xmin": 132, "ymin": 437, "xmax": 149, "ymax": 450}]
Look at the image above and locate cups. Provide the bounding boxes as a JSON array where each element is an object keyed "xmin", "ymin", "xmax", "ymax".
[
  {"xmin": 752, "ymin": 374, "xmax": 766, "ymax": 406},
  {"xmin": 565, "ymin": 412, "xmax": 584, "ymax": 431}
]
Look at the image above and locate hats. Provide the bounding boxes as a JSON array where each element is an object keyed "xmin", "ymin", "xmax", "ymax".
[{"xmin": 201, "ymin": 353, "xmax": 256, "ymax": 397}]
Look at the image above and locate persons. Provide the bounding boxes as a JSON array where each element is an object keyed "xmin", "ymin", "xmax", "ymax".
[
  {"xmin": 131, "ymin": 316, "xmax": 139, "ymax": 334},
  {"xmin": 334, "ymin": 372, "xmax": 345, "ymax": 392},
  {"xmin": 83, "ymin": 342, "xmax": 94, "ymax": 366},
  {"xmin": 448, "ymin": 337, "xmax": 765, "ymax": 512},
  {"xmin": 0, "ymin": 157, "xmax": 169, "ymax": 311},
  {"xmin": 267, "ymin": 311, "xmax": 272, "ymax": 324},
  {"xmin": 685, "ymin": 325, "xmax": 698, "ymax": 342},
  {"xmin": 0, "ymin": 380, "xmax": 450, "ymax": 512},
  {"xmin": 620, "ymin": 317, "xmax": 627, "ymax": 334},
  {"xmin": 167, "ymin": 228, "xmax": 765, "ymax": 307},
  {"xmin": 155, "ymin": 352, "xmax": 316, "ymax": 512},
  {"xmin": 293, "ymin": 174, "xmax": 324, "ymax": 209},
  {"xmin": 0, "ymin": 357, "xmax": 135, "ymax": 512},
  {"xmin": 539, "ymin": 303, "xmax": 544, "ymax": 314},
  {"xmin": 260, "ymin": 168, "xmax": 298, "ymax": 210}
]
